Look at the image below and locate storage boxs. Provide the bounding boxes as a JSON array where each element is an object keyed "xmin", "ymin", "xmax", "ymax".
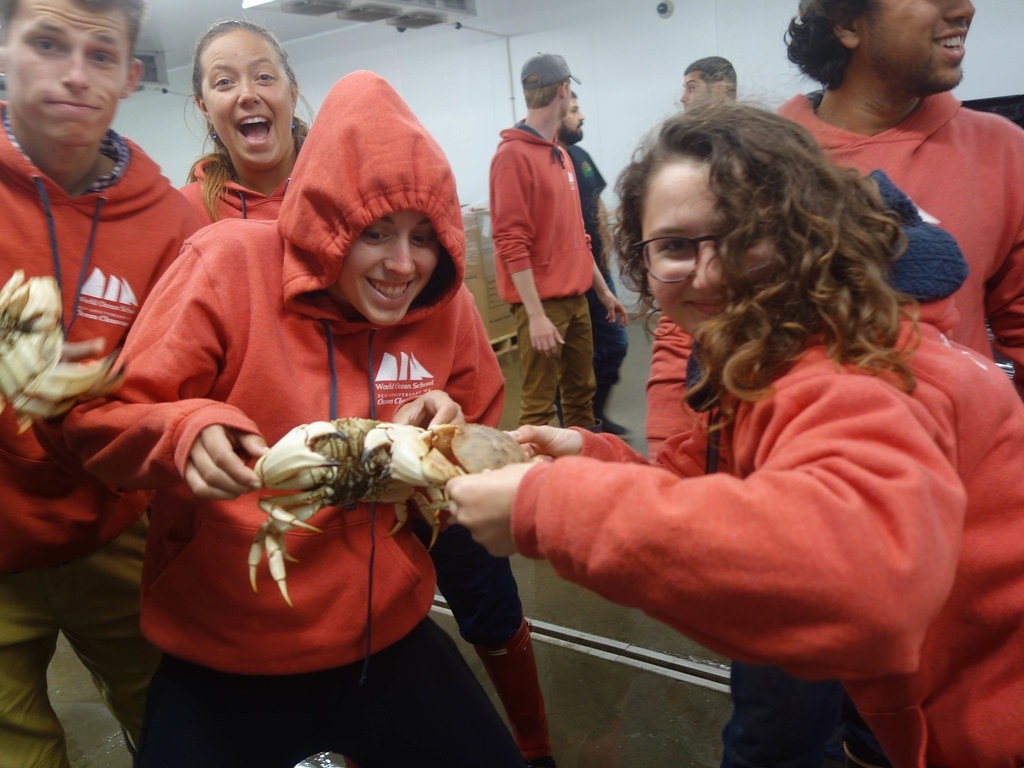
[{"xmin": 460, "ymin": 202, "xmax": 516, "ymax": 341}]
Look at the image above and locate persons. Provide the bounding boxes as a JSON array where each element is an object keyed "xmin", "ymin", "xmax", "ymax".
[
  {"xmin": 0, "ymin": 1, "xmax": 1024, "ymax": 767},
  {"xmin": 491, "ymin": 54, "xmax": 628, "ymax": 432}
]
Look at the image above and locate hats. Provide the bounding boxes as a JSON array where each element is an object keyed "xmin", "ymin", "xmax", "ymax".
[{"xmin": 521, "ymin": 52, "xmax": 582, "ymax": 90}]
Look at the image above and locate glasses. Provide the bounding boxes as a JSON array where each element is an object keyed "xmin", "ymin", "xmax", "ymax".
[{"xmin": 633, "ymin": 234, "xmax": 734, "ymax": 283}]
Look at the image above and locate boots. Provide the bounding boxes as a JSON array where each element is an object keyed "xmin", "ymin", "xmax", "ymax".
[{"xmin": 588, "ymin": 418, "xmax": 604, "ymax": 434}]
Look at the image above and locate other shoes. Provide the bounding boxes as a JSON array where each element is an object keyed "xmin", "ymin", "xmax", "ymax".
[
  {"xmin": 599, "ymin": 416, "xmax": 630, "ymax": 436},
  {"xmin": 527, "ymin": 755, "xmax": 557, "ymax": 768}
]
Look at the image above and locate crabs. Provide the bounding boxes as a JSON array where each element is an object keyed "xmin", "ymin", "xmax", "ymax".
[
  {"xmin": 248, "ymin": 416, "xmax": 532, "ymax": 608},
  {"xmin": 0, "ymin": 269, "xmax": 129, "ymax": 420}
]
